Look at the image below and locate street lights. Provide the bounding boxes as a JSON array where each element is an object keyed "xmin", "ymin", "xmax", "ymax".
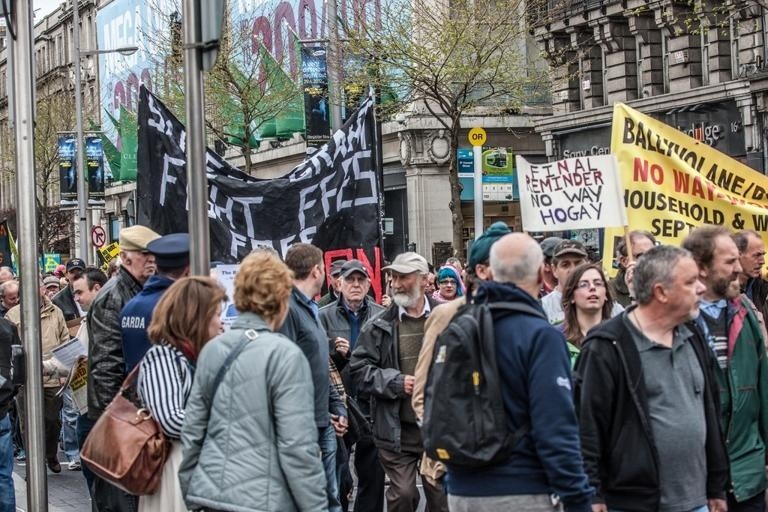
[{"xmin": 76, "ymin": 45, "xmax": 138, "ymax": 268}]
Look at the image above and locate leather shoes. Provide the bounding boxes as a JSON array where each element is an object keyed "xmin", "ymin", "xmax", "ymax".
[{"xmin": 48, "ymin": 459, "xmax": 60, "ymax": 473}]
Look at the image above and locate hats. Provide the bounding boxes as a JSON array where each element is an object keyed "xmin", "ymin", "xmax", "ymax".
[
  {"xmin": 118, "ymin": 225, "xmax": 161, "ymax": 250},
  {"xmin": 329, "ymin": 251, "xmax": 431, "ymax": 282},
  {"xmin": 43, "ymin": 276, "xmax": 59, "ymax": 289},
  {"xmin": 66, "ymin": 258, "xmax": 85, "ymax": 271},
  {"xmin": 539, "ymin": 237, "xmax": 586, "ymax": 257},
  {"xmin": 146, "ymin": 233, "xmax": 190, "ymax": 267}
]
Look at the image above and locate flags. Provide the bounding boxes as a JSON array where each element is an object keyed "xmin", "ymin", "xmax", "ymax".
[
  {"xmin": 88, "ymin": 120, "xmax": 121, "ymax": 182},
  {"xmin": 261, "ymin": 46, "xmax": 306, "ymax": 137},
  {"xmin": 228, "ymin": 64, "xmax": 292, "ymax": 139},
  {"xmin": 169, "ymin": 79, "xmax": 187, "ymax": 126},
  {"xmin": 118, "ymin": 105, "xmax": 138, "ymax": 182},
  {"xmin": 105, "ymin": 109, "xmax": 123, "ymax": 137},
  {"xmin": 211, "ymin": 82, "xmax": 258, "ymax": 149}
]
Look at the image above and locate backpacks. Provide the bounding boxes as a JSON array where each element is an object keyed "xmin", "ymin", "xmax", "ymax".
[{"xmin": 422, "ymin": 301, "xmax": 546, "ymax": 470}]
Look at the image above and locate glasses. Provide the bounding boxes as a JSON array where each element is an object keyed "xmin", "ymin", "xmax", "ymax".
[{"xmin": 577, "ymin": 280, "xmax": 604, "ymax": 288}]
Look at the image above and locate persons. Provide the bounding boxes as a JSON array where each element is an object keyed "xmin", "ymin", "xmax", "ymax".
[{"xmin": 0, "ymin": 220, "xmax": 767, "ymax": 511}]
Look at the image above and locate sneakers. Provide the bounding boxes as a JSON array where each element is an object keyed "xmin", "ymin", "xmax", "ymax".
[{"xmin": 68, "ymin": 460, "xmax": 81, "ymax": 471}]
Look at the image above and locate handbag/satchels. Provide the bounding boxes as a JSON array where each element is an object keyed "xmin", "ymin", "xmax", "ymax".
[{"xmin": 78, "ymin": 396, "xmax": 171, "ymax": 496}]
